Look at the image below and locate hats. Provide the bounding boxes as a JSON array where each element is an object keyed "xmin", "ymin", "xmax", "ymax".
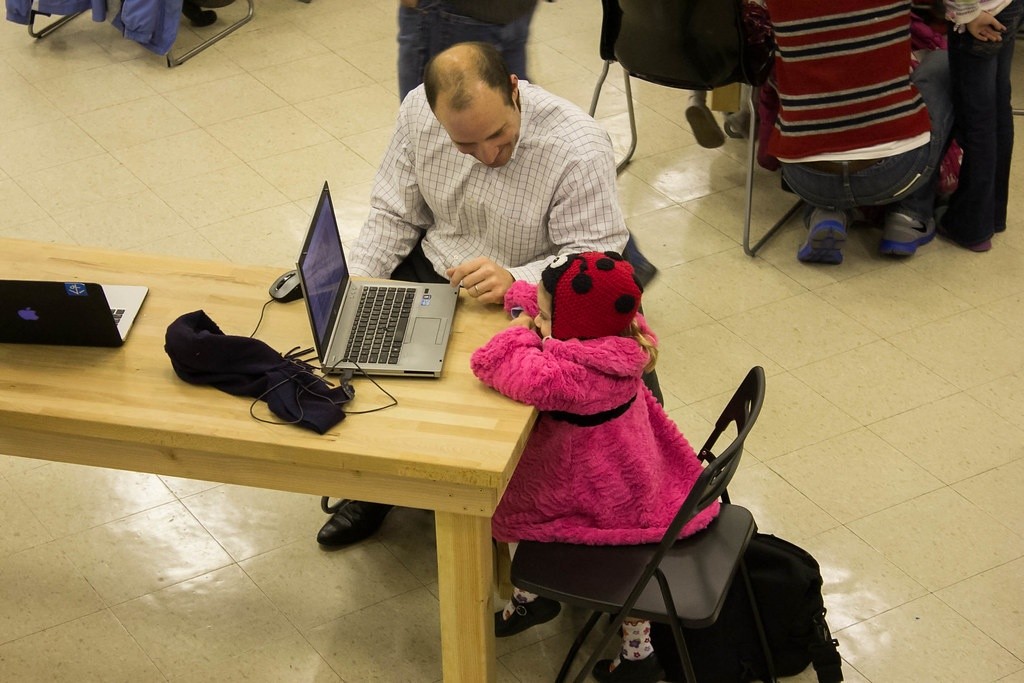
[{"xmin": 542, "ymin": 250, "xmax": 644, "ymax": 343}]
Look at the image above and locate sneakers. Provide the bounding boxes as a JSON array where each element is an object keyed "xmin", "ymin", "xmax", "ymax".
[
  {"xmin": 801, "ymin": 208, "xmax": 846, "ymax": 265},
  {"xmin": 880, "ymin": 210, "xmax": 938, "ymax": 254}
]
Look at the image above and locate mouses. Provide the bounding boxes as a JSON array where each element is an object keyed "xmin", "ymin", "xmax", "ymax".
[{"xmin": 269, "ymin": 270, "xmax": 304, "ymax": 303}]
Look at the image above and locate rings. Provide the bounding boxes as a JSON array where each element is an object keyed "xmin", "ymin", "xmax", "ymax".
[{"xmin": 474, "ymin": 285, "xmax": 482, "ymax": 295}]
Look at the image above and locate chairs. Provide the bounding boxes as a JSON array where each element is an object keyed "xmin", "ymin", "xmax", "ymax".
[
  {"xmin": 5, "ymin": 0, "xmax": 255, "ymax": 69},
  {"xmin": 589, "ymin": 0, "xmax": 801, "ymax": 254},
  {"xmin": 509, "ymin": 364, "xmax": 765, "ymax": 683}
]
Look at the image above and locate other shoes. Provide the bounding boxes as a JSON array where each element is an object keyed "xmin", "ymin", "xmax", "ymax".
[
  {"xmin": 938, "ymin": 204, "xmax": 1007, "ymax": 252},
  {"xmin": 593, "ymin": 651, "xmax": 658, "ymax": 683},
  {"xmin": 686, "ymin": 97, "xmax": 725, "ymax": 149},
  {"xmin": 495, "ymin": 595, "xmax": 561, "ymax": 637},
  {"xmin": 317, "ymin": 500, "xmax": 395, "ymax": 546},
  {"xmin": 729, "ymin": 110, "xmax": 758, "ymax": 148}
]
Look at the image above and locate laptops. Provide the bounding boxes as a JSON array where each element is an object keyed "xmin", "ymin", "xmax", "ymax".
[
  {"xmin": 295, "ymin": 181, "xmax": 460, "ymax": 377},
  {"xmin": 0, "ymin": 279, "xmax": 149, "ymax": 348}
]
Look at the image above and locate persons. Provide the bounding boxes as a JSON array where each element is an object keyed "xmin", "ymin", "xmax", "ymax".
[
  {"xmin": 933, "ymin": 0, "xmax": 1024, "ymax": 253},
  {"xmin": 396, "ymin": 0, "xmax": 539, "ymax": 103},
  {"xmin": 615, "ymin": 0, "xmax": 775, "ymax": 148},
  {"xmin": 317, "ymin": 42, "xmax": 631, "ymax": 546},
  {"xmin": 469, "ymin": 251, "xmax": 719, "ymax": 683},
  {"xmin": 766, "ymin": 0, "xmax": 954, "ymax": 265}
]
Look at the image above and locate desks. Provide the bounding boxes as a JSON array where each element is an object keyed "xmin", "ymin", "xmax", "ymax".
[{"xmin": 0, "ymin": 236, "xmax": 541, "ymax": 683}]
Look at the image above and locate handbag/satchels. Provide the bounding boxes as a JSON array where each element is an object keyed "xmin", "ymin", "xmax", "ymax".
[{"xmin": 623, "ymin": 533, "xmax": 845, "ymax": 683}]
